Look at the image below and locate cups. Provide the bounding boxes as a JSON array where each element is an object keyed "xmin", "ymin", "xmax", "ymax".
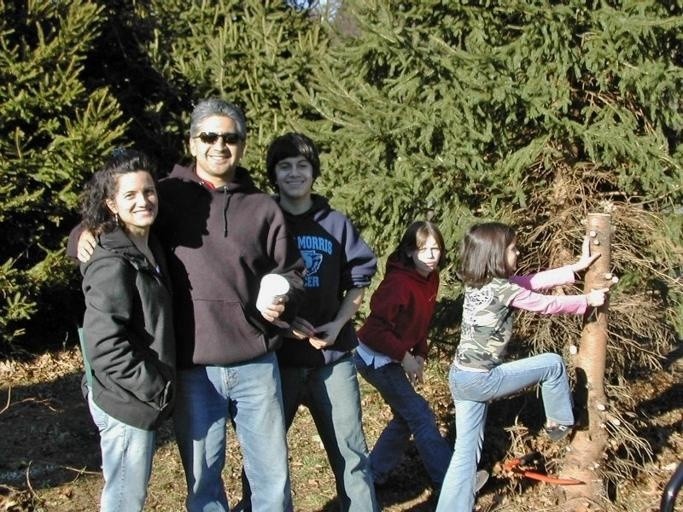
[{"xmin": 254, "ymin": 273, "xmax": 291, "ymax": 315}]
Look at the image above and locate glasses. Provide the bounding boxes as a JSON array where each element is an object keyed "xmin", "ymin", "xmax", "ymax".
[{"xmin": 191, "ymin": 132, "xmax": 242, "ymax": 144}]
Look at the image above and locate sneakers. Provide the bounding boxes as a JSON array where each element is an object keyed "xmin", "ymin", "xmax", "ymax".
[
  {"xmin": 476, "ymin": 470, "xmax": 489, "ymax": 491},
  {"xmin": 544, "ymin": 409, "xmax": 578, "ymax": 442},
  {"xmin": 375, "ymin": 474, "xmax": 407, "ymax": 491}
]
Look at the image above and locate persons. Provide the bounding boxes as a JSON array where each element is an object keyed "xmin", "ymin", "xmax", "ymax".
[
  {"xmin": 61, "ymin": 98, "xmax": 311, "ymax": 511},
  {"xmin": 76, "ymin": 146, "xmax": 182, "ymax": 512},
  {"xmin": 431, "ymin": 222, "xmax": 610, "ymax": 512},
  {"xmin": 351, "ymin": 218, "xmax": 453, "ymax": 490},
  {"xmin": 253, "ymin": 131, "xmax": 379, "ymax": 512}
]
